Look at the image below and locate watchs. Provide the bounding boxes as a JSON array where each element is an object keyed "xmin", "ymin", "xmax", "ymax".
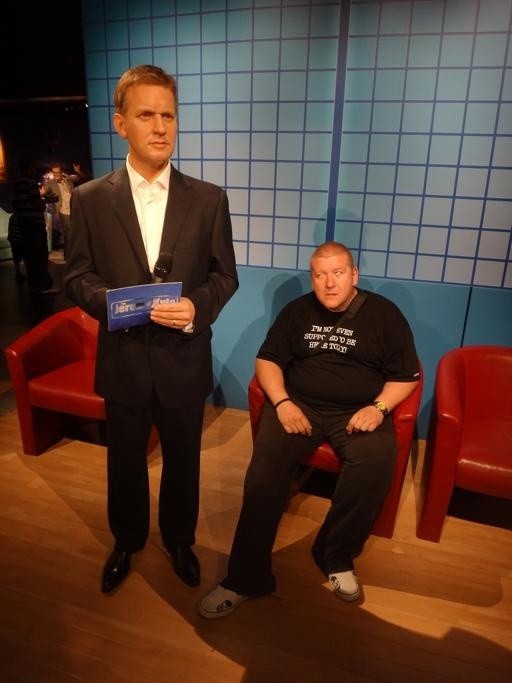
[{"xmin": 371, "ymin": 399, "xmax": 388, "ymax": 418}]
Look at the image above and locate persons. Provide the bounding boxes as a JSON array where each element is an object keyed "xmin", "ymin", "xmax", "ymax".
[
  {"xmin": 1, "ymin": 161, "xmax": 88, "ymax": 290},
  {"xmin": 62, "ymin": 62, "xmax": 240, "ymax": 596},
  {"xmin": 190, "ymin": 238, "xmax": 421, "ymax": 616}
]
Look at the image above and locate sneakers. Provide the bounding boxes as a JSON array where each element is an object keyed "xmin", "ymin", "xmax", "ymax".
[
  {"xmin": 327, "ymin": 566, "xmax": 362, "ymax": 604},
  {"xmin": 197, "ymin": 582, "xmax": 246, "ymax": 621}
]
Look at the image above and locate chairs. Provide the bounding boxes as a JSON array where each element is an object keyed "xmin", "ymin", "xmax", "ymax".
[
  {"xmin": 246, "ymin": 354, "xmax": 422, "ymax": 541},
  {"xmin": 8, "ymin": 305, "xmax": 162, "ymax": 459},
  {"xmin": 417, "ymin": 346, "xmax": 512, "ymax": 543}
]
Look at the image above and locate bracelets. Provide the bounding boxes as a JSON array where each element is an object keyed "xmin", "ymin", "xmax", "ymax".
[{"xmin": 275, "ymin": 397, "xmax": 290, "ymax": 408}]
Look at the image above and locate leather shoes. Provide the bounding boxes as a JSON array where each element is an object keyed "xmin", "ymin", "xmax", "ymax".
[
  {"xmin": 98, "ymin": 535, "xmax": 148, "ymax": 598},
  {"xmin": 161, "ymin": 537, "xmax": 202, "ymax": 589}
]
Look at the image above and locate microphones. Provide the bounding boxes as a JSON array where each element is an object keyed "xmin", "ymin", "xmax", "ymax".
[{"xmin": 151, "ymin": 252, "xmax": 174, "ymax": 284}]
